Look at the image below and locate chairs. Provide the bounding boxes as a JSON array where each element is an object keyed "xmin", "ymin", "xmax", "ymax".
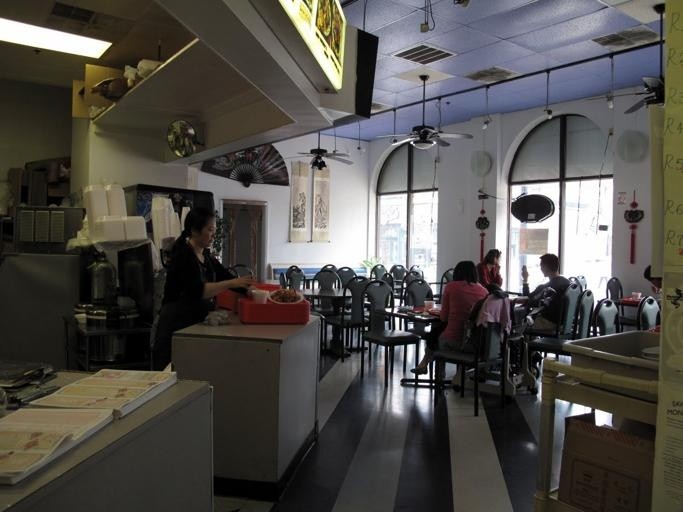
[
  {"xmin": 431, "ymin": 293, "xmax": 509, "ymax": 416},
  {"xmin": 360, "ymin": 280, "xmax": 419, "ymax": 390},
  {"xmin": 324, "ymin": 276, "xmax": 371, "ymax": 361},
  {"xmin": 606, "ymin": 276, "xmax": 625, "ymax": 326},
  {"xmin": 527, "ymin": 290, "xmax": 594, "ymax": 392},
  {"xmin": 637, "ymin": 294, "xmax": 660, "ymax": 328},
  {"xmin": 278, "ymin": 264, "xmax": 424, "ymax": 340},
  {"xmin": 404, "ymin": 278, "xmax": 433, "ymax": 333},
  {"xmin": 593, "ymin": 298, "xmax": 621, "ymax": 336},
  {"xmin": 517, "ymin": 277, "xmax": 583, "ymax": 372}
]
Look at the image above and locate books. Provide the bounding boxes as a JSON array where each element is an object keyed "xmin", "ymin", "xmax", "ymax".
[{"xmin": 0, "ymin": 368, "xmax": 178, "ymax": 486}]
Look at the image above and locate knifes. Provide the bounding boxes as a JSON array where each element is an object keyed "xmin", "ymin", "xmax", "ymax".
[{"xmin": 20, "ymin": 384, "xmax": 59, "ymax": 402}]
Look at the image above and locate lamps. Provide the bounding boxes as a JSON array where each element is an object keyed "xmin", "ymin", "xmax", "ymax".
[
  {"xmin": 333, "ymin": 51, "xmax": 615, "ymax": 154},
  {"xmin": 310, "ymin": 154, "xmax": 327, "ymax": 171}
]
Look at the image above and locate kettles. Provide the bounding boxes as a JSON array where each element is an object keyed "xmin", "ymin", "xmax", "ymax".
[{"xmin": 90, "ymin": 253, "xmax": 117, "ymax": 305}]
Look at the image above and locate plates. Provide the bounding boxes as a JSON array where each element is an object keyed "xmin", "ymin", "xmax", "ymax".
[
  {"xmin": 269, "ymin": 289, "xmax": 304, "ymax": 305},
  {"xmin": 83, "ymin": 182, "xmax": 146, "ymax": 242}
]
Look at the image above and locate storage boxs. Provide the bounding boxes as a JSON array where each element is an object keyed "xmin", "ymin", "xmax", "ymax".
[{"xmin": 557, "ymin": 412, "xmax": 655, "ymax": 512}]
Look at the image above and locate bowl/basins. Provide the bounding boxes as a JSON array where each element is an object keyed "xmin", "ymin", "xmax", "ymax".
[{"xmin": 74, "ymin": 314, "xmax": 86, "ymax": 323}]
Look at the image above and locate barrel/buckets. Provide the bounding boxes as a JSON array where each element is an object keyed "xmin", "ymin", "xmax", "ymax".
[{"xmin": 83, "ymin": 252, "xmax": 118, "ymax": 302}]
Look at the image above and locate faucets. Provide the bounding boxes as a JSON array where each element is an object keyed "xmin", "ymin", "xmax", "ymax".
[{"xmin": 225, "ymin": 264, "xmax": 254, "ymax": 279}]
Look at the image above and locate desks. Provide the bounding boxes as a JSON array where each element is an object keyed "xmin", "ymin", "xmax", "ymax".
[
  {"xmin": 381, "ymin": 300, "xmax": 524, "ymax": 385},
  {"xmin": 62, "ymin": 314, "xmax": 158, "ymax": 371},
  {"xmin": 604, "ymin": 294, "xmax": 649, "ymax": 330}
]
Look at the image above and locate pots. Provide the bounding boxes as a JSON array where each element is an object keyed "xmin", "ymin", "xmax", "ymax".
[{"xmin": 84, "ymin": 310, "xmax": 140, "ymax": 332}]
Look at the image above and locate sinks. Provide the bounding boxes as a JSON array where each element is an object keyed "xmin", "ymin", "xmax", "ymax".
[{"xmin": 562, "ymin": 330, "xmax": 661, "ymax": 381}]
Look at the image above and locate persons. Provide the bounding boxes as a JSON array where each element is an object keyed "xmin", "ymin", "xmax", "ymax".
[
  {"xmin": 475, "ymin": 248, "xmax": 503, "ymax": 288},
  {"xmin": 514, "ymin": 253, "xmax": 571, "ymax": 331},
  {"xmin": 150, "ymin": 206, "xmax": 259, "ymax": 371},
  {"xmin": 410, "ymin": 260, "xmax": 490, "ymax": 375},
  {"xmin": 644, "ymin": 265, "xmax": 663, "ymax": 296}
]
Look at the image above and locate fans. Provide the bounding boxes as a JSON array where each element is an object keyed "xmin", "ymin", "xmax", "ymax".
[
  {"xmin": 285, "ymin": 130, "xmax": 354, "ymax": 166},
  {"xmin": 585, "ymin": 3, "xmax": 665, "ymax": 115}
]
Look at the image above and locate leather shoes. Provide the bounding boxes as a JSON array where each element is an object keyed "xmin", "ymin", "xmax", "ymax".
[
  {"xmin": 410, "ymin": 366, "xmax": 428, "ymax": 374},
  {"xmin": 469, "ymin": 374, "xmax": 486, "ymax": 383}
]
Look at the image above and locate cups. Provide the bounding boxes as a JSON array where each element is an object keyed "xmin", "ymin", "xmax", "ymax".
[
  {"xmin": 631, "ymin": 292, "xmax": 641, "ymax": 299},
  {"xmin": 252, "ymin": 288, "xmax": 268, "ymax": 305},
  {"xmin": 150, "ymin": 196, "xmax": 181, "ymax": 245},
  {"xmin": 231, "ymin": 295, "xmax": 239, "ymax": 312},
  {"xmin": 423, "ymin": 300, "xmax": 435, "ymax": 309}
]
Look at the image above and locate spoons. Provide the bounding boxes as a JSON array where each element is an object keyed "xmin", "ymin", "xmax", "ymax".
[{"xmin": 10, "ymin": 366, "xmax": 54, "ymax": 389}]
